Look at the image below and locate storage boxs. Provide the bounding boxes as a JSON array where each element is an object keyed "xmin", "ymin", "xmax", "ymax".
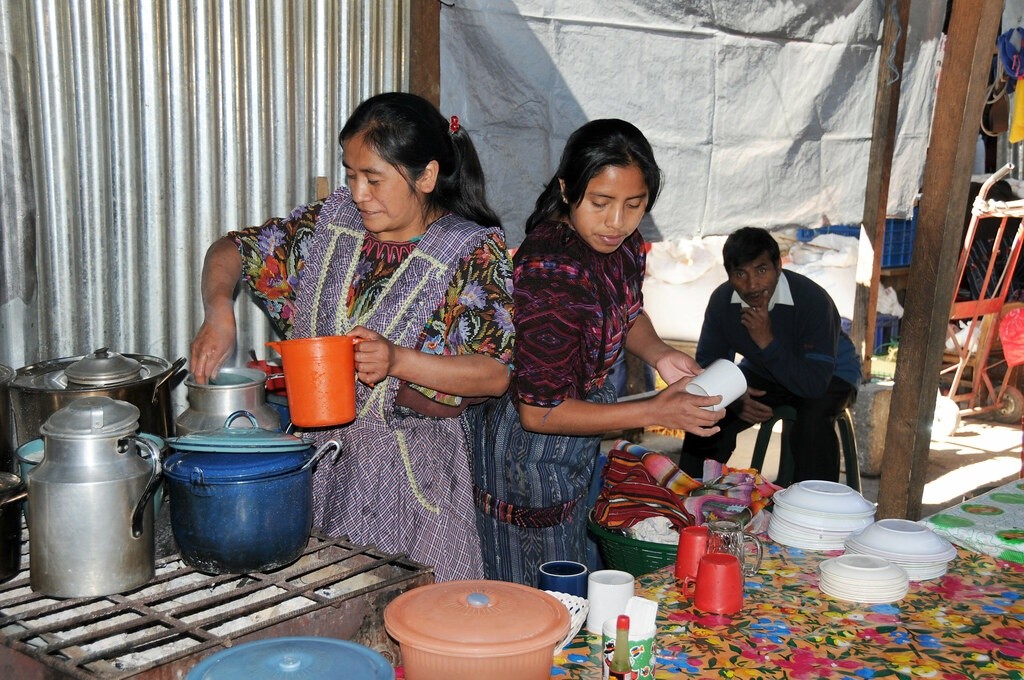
[
  {"xmin": 795, "ymin": 205, "xmax": 918, "ymax": 269},
  {"xmin": 843, "ymin": 315, "xmax": 897, "ymax": 357}
]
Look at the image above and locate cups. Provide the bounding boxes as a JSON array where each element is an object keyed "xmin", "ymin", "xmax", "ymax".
[
  {"xmin": 539, "ymin": 561, "xmax": 587, "ymax": 602},
  {"xmin": 705, "ymin": 521, "xmax": 763, "ymax": 580},
  {"xmin": 695, "ymin": 556, "xmax": 745, "ymax": 614},
  {"xmin": 676, "ymin": 526, "xmax": 720, "ymax": 582},
  {"xmin": 586, "ymin": 568, "xmax": 634, "ymax": 635},
  {"xmin": 687, "ymin": 358, "xmax": 748, "ymax": 413},
  {"xmin": 602, "ymin": 617, "xmax": 657, "ymax": 680}
]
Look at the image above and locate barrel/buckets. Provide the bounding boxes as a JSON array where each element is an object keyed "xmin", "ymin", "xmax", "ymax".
[
  {"xmin": 27, "ymin": 395, "xmax": 166, "ymax": 599},
  {"xmin": 175, "ymin": 366, "xmax": 282, "ymax": 438},
  {"xmin": 382, "ymin": 580, "xmax": 572, "ymax": 680},
  {"xmin": 182, "ymin": 634, "xmax": 399, "ymax": 680}
]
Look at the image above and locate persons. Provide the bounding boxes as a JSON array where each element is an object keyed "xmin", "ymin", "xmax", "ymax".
[
  {"xmin": 680, "ymin": 226, "xmax": 864, "ymax": 493},
  {"xmin": 466, "ymin": 119, "xmax": 726, "ymax": 594},
  {"xmin": 189, "ymin": 93, "xmax": 516, "ymax": 585}
]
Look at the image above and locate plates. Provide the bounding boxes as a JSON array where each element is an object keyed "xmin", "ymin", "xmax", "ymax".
[{"xmin": 768, "ymin": 479, "xmax": 958, "ymax": 603}]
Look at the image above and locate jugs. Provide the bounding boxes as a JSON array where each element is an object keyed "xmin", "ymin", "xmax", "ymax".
[{"xmin": 265, "ymin": 335, "xmax": 373, "ymax": 427}]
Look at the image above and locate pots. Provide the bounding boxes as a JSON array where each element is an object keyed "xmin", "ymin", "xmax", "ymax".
[
  {"xmin": 1, "ymin": 353, "xmax": 187, "ymax": 449},
  {"xmin": 0, "ymin": 362, "xmax": 14, "ymax": 471},
  {"xmin": 162, "ymin": 411, "xmax": 313, "ymax": 575},
  {"xmin": 0, "ymin": 470, "xmax": 25, "ymax": 584},
  {"xmin": 14, "ymin": 432, "xmax": 167, "ymax": 518}
]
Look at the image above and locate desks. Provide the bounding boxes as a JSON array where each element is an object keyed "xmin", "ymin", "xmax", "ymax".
[
  {"xmin": 552, "ymin": 514, "xmax": 1024, "ymax": 680},
  {"xmin": 878, "ymin": 265, "xmax": 912, "ymax": 304}
]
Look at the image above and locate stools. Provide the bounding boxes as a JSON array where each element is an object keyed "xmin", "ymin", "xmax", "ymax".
[{"xmin": 750, "ymin": 403, "xmax": 861, "ymax": 497}]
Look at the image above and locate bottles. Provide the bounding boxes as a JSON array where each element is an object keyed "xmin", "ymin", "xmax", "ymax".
[{"xmin": 609, "ymin": 614, "xmax": 632, "ymax": 680}]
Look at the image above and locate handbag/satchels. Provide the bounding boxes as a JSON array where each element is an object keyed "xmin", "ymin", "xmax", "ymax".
[{"xmin": 594, "ymin": 440, "xmax": 782, "ymax": 530}]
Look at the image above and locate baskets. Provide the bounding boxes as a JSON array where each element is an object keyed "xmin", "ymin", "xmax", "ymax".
[{"xmin": 588, "ymin": 506, "xmax": 678, "ymax": 576}]
